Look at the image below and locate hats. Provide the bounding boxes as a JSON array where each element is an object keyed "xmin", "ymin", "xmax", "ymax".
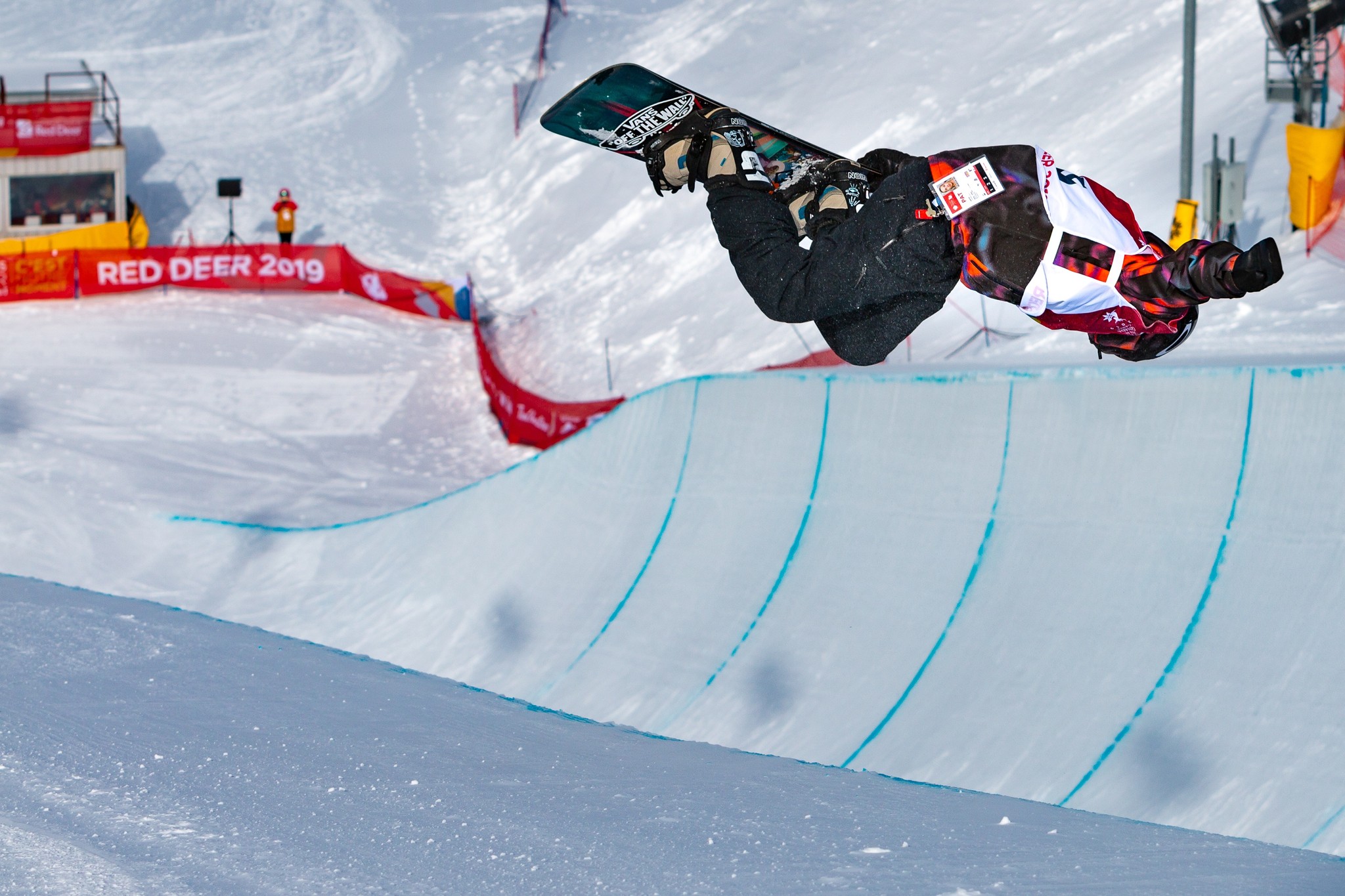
[{"xmin": 280, "ymin": 189, "xmax": 289, "ymax": 197}]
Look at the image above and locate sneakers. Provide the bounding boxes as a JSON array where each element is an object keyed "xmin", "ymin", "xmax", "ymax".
[
  {"xmin": 786, "ymin": 159, "xmax": 870, "ymax": 237},
  {"xmin": 658, "ymin": 107, "xmax": 775, "ymax": 191}
]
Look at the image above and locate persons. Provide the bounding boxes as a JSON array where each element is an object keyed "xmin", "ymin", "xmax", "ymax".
[
  {"xmin": 126, "ymin": 195, "xmax": 150, "ymax": 247},
  {"xmin": 273, "ymin": 188, "xmax": 297, "ymax": 243},
  {"xmin": 643, "ymin": 105, "xmax": 1285, "ymax": 365}
]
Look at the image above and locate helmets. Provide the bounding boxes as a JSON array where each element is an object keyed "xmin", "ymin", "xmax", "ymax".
[{"xmin": 1088, "ymin": 304, "xmax": 1199, "ymax": 362}]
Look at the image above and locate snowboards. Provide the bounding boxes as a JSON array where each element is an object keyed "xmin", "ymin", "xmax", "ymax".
[{"xmin": 540, "ymin": 62, "xmax": 883, "ymax": 206}]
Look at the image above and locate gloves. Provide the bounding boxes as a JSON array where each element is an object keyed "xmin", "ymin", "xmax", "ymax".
[
  {"xmin": 857, "ymin": 149, "xmax": 926, "ymax": 193},
  {"xmin": 1231, "ymin": 238, "xmax": 1283, "ymax": 292}
]
